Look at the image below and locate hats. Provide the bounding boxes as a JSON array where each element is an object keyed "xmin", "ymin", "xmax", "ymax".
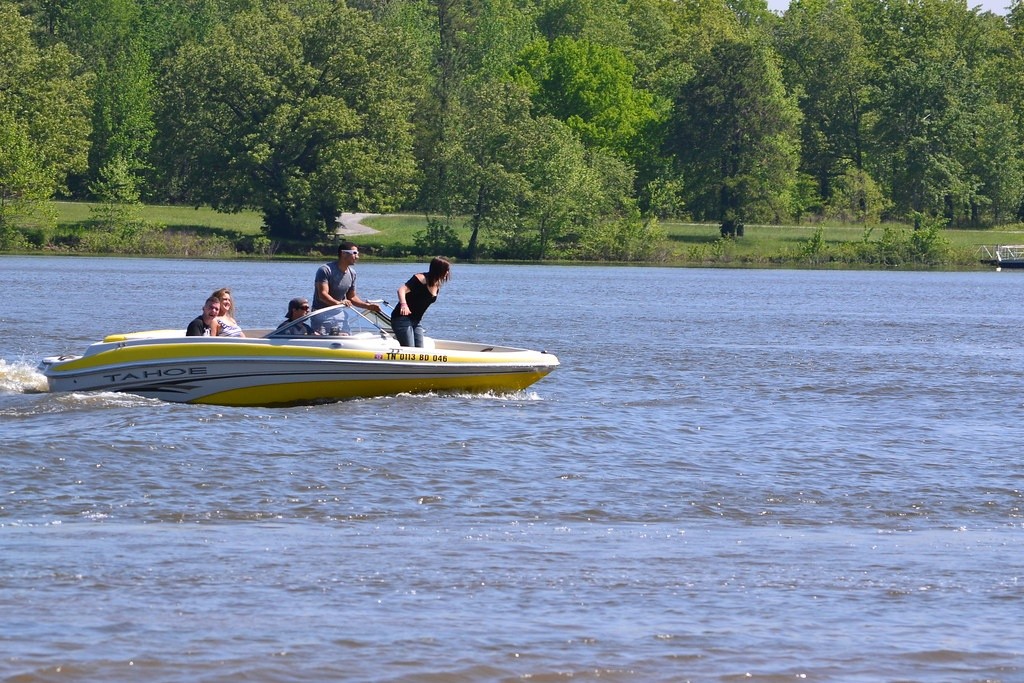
[{"xmin": 285, "ymin": 298, "xmax": 308, "ymax": 317}]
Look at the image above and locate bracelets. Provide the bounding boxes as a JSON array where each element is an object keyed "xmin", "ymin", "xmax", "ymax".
[{"xmin": 401, "ymin": 303, "xmax": 408, "ymax": 306}]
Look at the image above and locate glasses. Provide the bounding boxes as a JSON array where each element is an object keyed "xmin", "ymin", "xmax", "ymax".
[
  {"xmin": 299, "ymin": 305, "xmax": 309, "ymax": 313},
  {"xmin": 342, "ymin": 249, "xmax": 359, "ymax": 255}
]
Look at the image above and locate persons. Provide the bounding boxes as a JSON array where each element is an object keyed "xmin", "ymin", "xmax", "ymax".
[
  {"xmin": 391, "ymin": 255, "xmax": 451, "ymax": 348},
  {"xmin": 310, "ymin": 241, "xmax": 381, "ymax": 335},
  {"xmin": 276, "ymin": 297, "xmax": 321, "ymax": 335},
  {"xmin": 210, "ymin": 288, "xmax": 245, "ymax": 338},
  {"xmin": 186, "ymin": 296, "xmax": 220, "ymax": 336}
]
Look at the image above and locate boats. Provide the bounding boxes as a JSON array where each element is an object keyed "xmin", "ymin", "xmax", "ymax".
[{"xmin": 36, "ymin": 299, "xmax": 561, "ymax": 407}]
